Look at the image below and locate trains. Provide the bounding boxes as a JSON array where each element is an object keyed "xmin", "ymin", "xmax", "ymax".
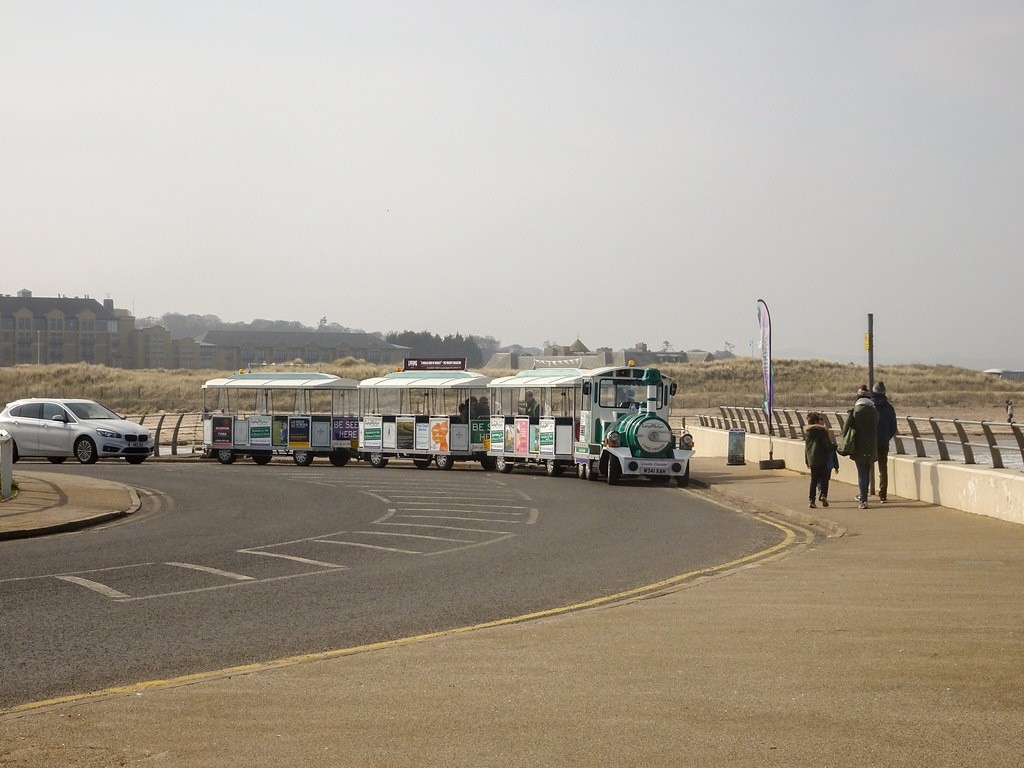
[{"xmin": 201, "ymin": 358, "xmax": 695, "ymax": 487}]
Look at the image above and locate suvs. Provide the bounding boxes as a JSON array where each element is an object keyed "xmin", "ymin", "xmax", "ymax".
[{"xmin": 0, "ymin": 397, "xmax": 156, "ymax": 465}]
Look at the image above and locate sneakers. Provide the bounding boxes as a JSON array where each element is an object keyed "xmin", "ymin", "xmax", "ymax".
[
  {"xmin": 855, "ymin": 494, "xmax": 862, "ymax": 501},
  {"xmin": 858, "ymin": 501, "xmax": 868, "ymax": 508},
  {"xmin": 880, "ymin": 496, "xmax": 888, "ymax": 504}
]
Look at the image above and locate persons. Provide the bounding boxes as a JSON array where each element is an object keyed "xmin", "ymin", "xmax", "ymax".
[
  {"xmin": 842, "ymin": 385, "xmax": 879, "ymax": 509},
  {"xmin": 870, "ymin": 381, "xmax": 898, "ymax": 504},
  {"xmin": 804, "ymin": 412, "xmax": 839, "ymax": 508},
  {"xmin": 457, "ymin": 395, "xmax": 490, "ymax": 424},
  {"xmin": 525, "ymin": 390, "xmax": 543, "ymax": 416}
]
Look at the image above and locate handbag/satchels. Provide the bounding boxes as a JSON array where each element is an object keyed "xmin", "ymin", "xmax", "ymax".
[{"xmin": 836, "ymin": 408, "xmax": 856, "ymax": 456}]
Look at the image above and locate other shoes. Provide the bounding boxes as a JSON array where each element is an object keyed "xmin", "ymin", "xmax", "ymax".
[
  {"xmin": 821, "ymin": 494, "xmax": 830, "ymax": 508},
  {"xmin": 818, "ymin": 490, "xmax": 823, "ymax": 501},
  {"xmin": 809, "ymin": 500, "xmax": 817, "ymax": 508}
]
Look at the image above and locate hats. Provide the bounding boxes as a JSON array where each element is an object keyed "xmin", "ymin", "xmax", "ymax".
[
  {"xmin": 858, "ymin": 385, "xmax": 871, "ymax": 397},
  {"xmin": 872, "ymin": 381, "xmax": 887, "ymax": 394}
]
